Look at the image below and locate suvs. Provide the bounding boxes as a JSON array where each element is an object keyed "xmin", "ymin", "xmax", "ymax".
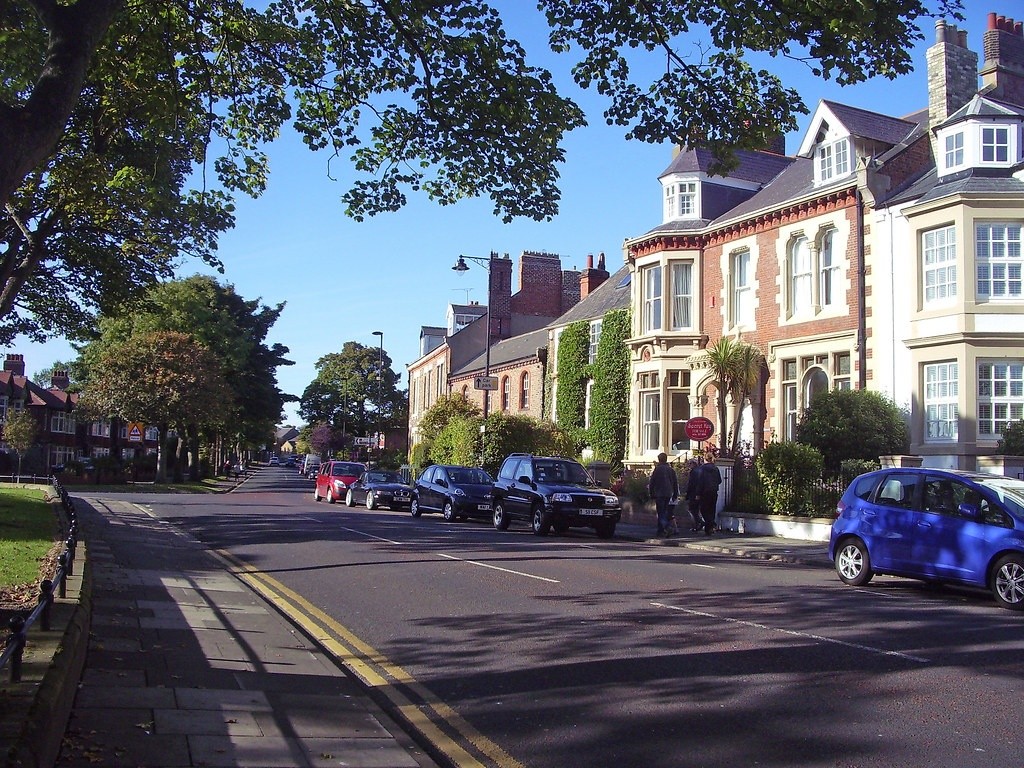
[{"xmin": 491, "ymin": 452, "xmax": 624, "ymax": 540}]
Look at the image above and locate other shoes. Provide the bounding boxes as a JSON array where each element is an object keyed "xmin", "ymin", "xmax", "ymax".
[
  {"xmin": 690, "ymin": 522, "xmax": 705, "ymax": 531},
  {"xmin": 675, "ymin": 529, "xmax": 679, "ymax": 534},
  {"xmin": 708, "ymin": 523, "xmax": 717, "ymax": 534},
  {"xmin": 666, "ymin": 530, "xmax": 674, "ymax": 538},
  {"xmin": 656, "ymin": 533, "xmax": 663, "ymax": 537}
]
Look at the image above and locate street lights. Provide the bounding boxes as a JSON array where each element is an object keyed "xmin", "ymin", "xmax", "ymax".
[
  {"xmin": 371, "ymin": 331, "xmax": 383, "ymax": 465},
  {"xmin": 334, "ymin": 377, "xmax": 350, "ymax": 463},
  {"xmin": 452, "ymin": 255, "xmax": 492, "ymax": 463}
]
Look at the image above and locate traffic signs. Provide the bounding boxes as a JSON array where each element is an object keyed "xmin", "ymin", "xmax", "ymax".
[
  {"xmin": 474, "ymin": 376, "xmax": 499, "ymax": 390},
  {"xmin": 354, "ymin": 437, "xmax": 377, "ymax": 447}
]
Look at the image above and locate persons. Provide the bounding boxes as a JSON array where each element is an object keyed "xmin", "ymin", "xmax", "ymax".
[
  {"xmin": 242, "ymin": 459, "xmax": 248, "ymax": 476},
  {"xmin": 648, "ymin": 453, "xmax": 680, "ymax": 538},
  {"xmin": 224, "ymin": 461, "xmax": 232, "ymax": 479},
  {"xmin": 234, "ymin": 462, "xmax": 240, "ymax": 481},
  {"xmin": 685, "ymin": 453, "xmax": 722, "ymax": 536}
]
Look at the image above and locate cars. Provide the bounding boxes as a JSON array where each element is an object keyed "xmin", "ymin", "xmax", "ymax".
[
  {"xmin": 286, "ymin": 454, "xmax": 325, "ymax": 480},
  {"xmin": 344, "ymin": 470, "xmax": 413, "ymax": 512},
  {"xmin": 313, "ymin": 462, "xmax": 368, "ymax": 505},
  {"xmin": 409, "ymin": 464, "xmax": 497, "ymax": 523},
  {"xmin": 829, "ymin": 468, "xmax": 1024, "ymax": 612},
  {"xmin": 268, "ymin": 456, "xmax": 280, "ymax": 467}
]
